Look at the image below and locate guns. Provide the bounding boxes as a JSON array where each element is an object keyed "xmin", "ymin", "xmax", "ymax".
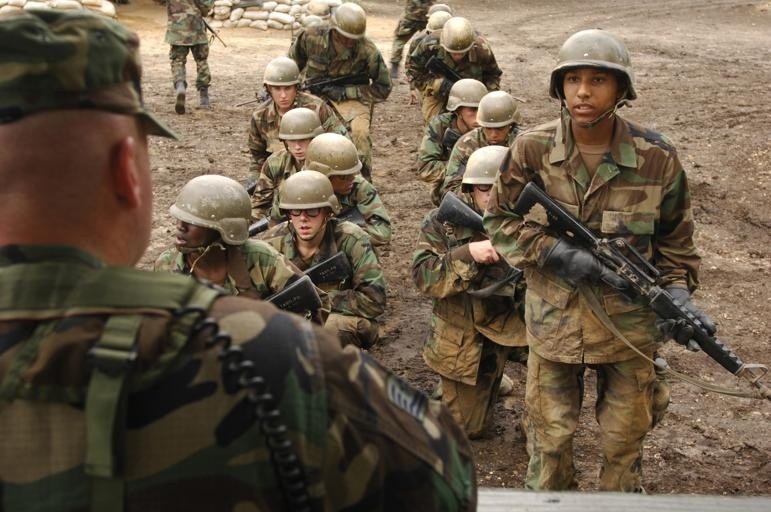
[
  {"xmin": 436, "ymin": 191, "xmax": 526, "ymax": 285},
  {"xmin": 515, "ymin": 181, "xmax": 771, "ymax": 400},
  {"xmin": 202, "ymin": 18, "xmax": 227, "ymax": 47},
  {"xmin": 424, "ymin": 55, "xmax": 463, "ymax": 83},
  {"xmin": 442, "ymin": 126, "xmax": 463, "ymax": 150},
  {"xmin": 250, "ymin": 206, "xmax": 368, "ymax": 237},
  {"xmin": 264, "ymin": 274, "xmax": 322, "ymax": 313},
  {"xmin": 236, "ymin": 71, "xmax": 369, "ymax": 107},
  {"xmin": 302, "ymin": 250, "xmax": 353, "ymax": 282}
]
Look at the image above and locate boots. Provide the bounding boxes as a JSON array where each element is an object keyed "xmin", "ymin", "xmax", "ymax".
[
  {"xmin": 199, "ymin": 87, "xmax": 210, "ymax": 108},
  {"xmin": 175, "ymin": 81, "xmax": 186, "ymax": 114}
]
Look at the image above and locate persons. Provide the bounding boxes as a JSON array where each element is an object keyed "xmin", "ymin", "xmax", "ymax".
[
  {"xmin": 0, "ymin": 10, "xmax": 477, "ymax": 512},
  {"xmin": 482, "ymin": 28, "xmax": 717, "ymax": 494},
  {"xmin": 151, "ymin": 1, "xmax": 533, "ymax": 443}
]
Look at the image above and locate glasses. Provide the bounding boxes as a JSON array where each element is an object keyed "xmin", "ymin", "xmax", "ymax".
[
  {"xmin": 290, "ymin": 207, "xmax": 321, "ymax": 218},
  {"xmin": 471, "ymin": 184, "xmax": 491, "ymax": 192}
]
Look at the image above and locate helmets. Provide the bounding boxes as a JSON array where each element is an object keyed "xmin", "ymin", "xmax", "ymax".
[
  {"xmin": 446, "ymin": 78, "xmax": 489, "ymax": 112},
  {"xmin": 304, "ymin": 132, "xmax": 363, "ymax": 178},
  {"xmin": 475, "ymin": 90, "xmax": 524, "ymax": 129},
  {"xmin": 278, "ymin": 107, "xmax": 324, "ymax": 140},
  {"xmin": 425, "ymin": 3, "xmax": 452, "ymax": 18},
  {"xmin": 548, "ymin": 29, "xmax": 638, "ymax": 100},
  {"xmin": 169, "ymin": 172, "xmax": 253, "ymax": 244},
  {"xmin": 425, "ymin": 10, "xmax": 452, "ymax": 32},
  {"xmin": 460, "ymin": 144, "xmax": 511, "ymax": 193},
  {"xmin": 329, "ymin": 1, "xmax": 367, "ymax": 39},
  {"xmin": 278, "ymin": 169, "xmax": 342, "ymax": 215},
  {"xmin": 440, "ymin": 16, "xmax": 477, "ymax": 52},
  {"xmin": 263, "ymin": 56, "xmax": 302, "ymax": 86}
]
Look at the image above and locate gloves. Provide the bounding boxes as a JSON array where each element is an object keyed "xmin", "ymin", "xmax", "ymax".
[
  {"xmin": 320, "ymin": 82, "xmax": 347, "ymax": 101},
  {"xmin": 540, "ymin": 238, "xmax": 631, "ymax": 293},
  {"xmin": 439, "ymin": 78, "xmax": 454, "ymax": 98},
  {"xmin": 654, "ymin": 285, "xmax": 717, "ymax": 352}
]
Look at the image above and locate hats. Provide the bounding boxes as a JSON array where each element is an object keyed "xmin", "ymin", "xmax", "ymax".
[{"xmin": 0, "ymin": 6, "xmax": 182, "ymax": 142}]
